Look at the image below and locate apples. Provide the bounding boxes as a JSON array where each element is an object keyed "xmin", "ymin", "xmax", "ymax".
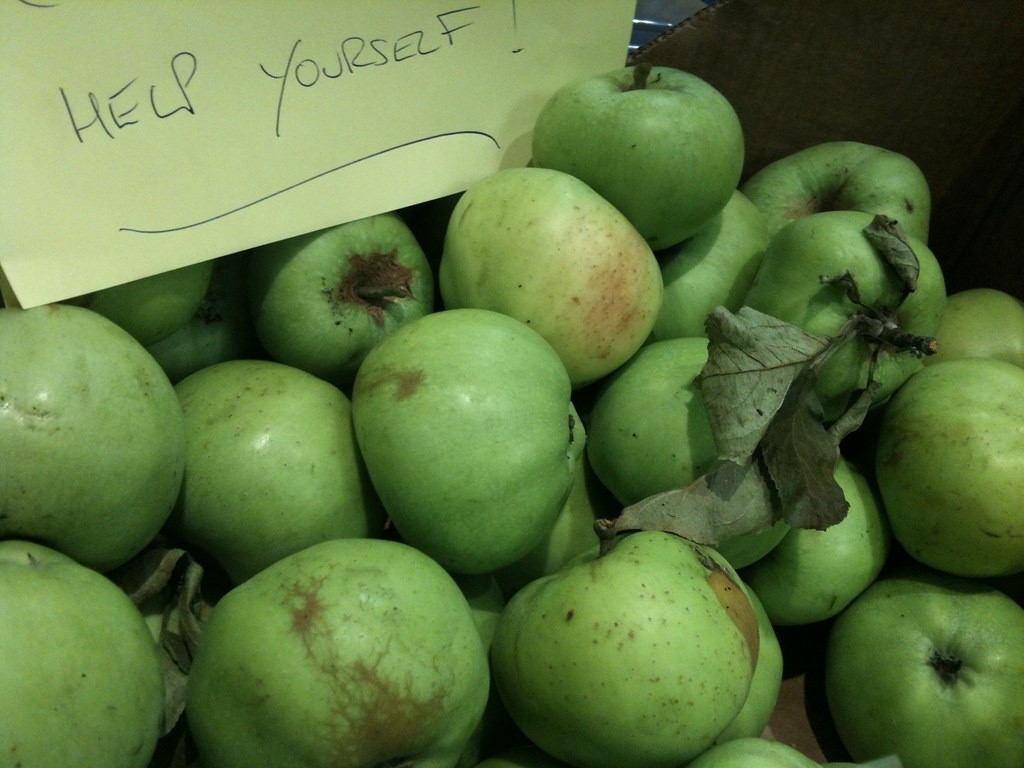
[{"xmin": 0, "ymin": 58, "xmax": 1024, "ymax": 767}]
